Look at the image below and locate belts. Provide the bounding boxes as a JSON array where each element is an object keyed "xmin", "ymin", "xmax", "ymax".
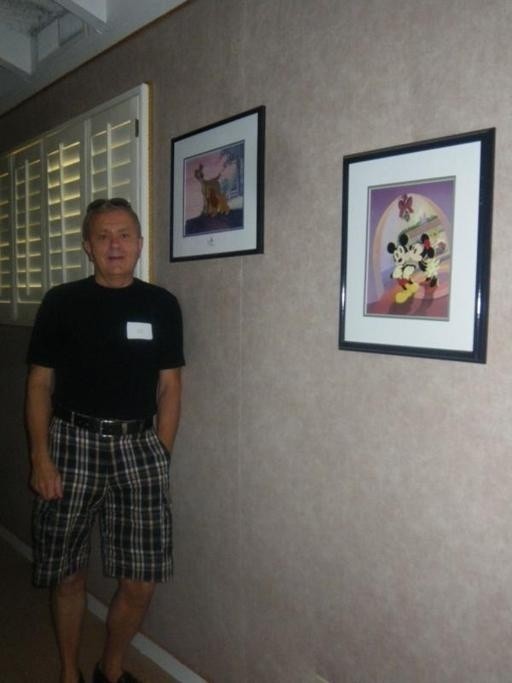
[{"xmin": 51, "ymin": 405, "xmax": 155, "ymax": 437}]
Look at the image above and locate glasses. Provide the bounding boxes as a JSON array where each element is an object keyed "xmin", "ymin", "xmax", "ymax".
[{"xmin": 84, "ymin": 196, "xmax": 131, "ymax": 241}]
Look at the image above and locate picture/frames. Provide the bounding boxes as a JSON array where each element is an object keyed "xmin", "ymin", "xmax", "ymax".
[
  {"xmin": 337, "ymin": 126, "xmax": 497, "ymax": 364},
  {"xmin": 169, "ymin": 105, "xmax": 267, "ymax": 263}
]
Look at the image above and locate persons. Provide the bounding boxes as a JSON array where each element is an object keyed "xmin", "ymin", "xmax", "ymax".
[{"xmin": 24, "ymin": 198, "xmax": 185, "ymax": 683}]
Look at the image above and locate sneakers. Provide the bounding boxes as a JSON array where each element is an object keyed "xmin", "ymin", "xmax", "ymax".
[{"xmin": 75, "ymin": 659, "xmax": 142, "ymax": 682}]
